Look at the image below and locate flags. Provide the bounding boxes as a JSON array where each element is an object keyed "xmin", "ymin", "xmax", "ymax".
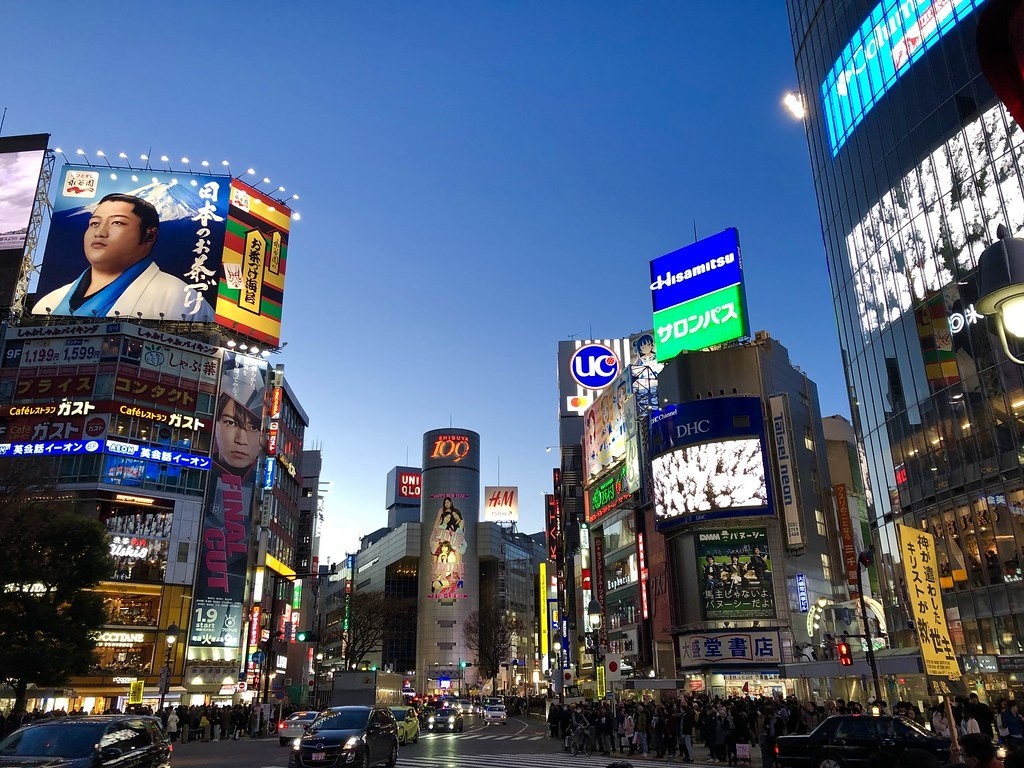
[
  {"xmin": 606, "ymin": 653, "xmax": 622, "ymax": 681},
  {"xmin": 563, "ymin": 669, "xmax": 573, "ymax": 685},
  {"xmin": 519, "ymin": 679, "xmax": 524, "ymax": 688}
]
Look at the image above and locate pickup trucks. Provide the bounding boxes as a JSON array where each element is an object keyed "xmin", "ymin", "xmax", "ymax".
[{"xmin": 483, "ymin": 704, "xmax": 506, "ymax": 726}]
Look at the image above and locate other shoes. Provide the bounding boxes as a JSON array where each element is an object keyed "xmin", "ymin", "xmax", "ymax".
[
  {"xmin": 714, "ymin": 759, "xmax": 719, "ymax": 763},
  {"xmin": 707, "ymin": 759, "xmax": 714, "ymax": 762},
  {"xmin": 600, "ymin": 748, "xmax": 694, "ymax": 761}
]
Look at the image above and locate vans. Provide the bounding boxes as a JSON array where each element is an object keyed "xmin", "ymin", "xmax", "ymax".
[
  {"xmin": 390, "ymin": 706, "xmax": 419, "ymax": 745},
  {"xmin": 288, "ymin": 705, "xmax": 398, "ymax": 768},
  {"xmin": 455, "ymin": 699, "xmax": 473, "ymax": 713},
  {"xmin": 0, "ymin": 713, "xmax": 174, "ymax": 768}
]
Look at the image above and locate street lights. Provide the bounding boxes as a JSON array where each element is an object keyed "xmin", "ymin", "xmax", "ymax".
[
  {"xmin": 552, "ymin": 629, "xmax": 562, "ymax": 702},
  {"xmin": 857, "ymin": 549, "xmax": 882, "ymax": 703},
  {"xmin": 587, "ymin": 596, "xmax": 603, "ymax": 706},
  {"xmin": 514, "ymin": 656, "xmax": 530, "ymax": 719},
  {"xmin": 158, "ymin": 622, "xmax": 177, "ymax": 717}
]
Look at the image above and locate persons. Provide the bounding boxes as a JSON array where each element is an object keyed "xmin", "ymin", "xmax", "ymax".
[
  {"xmin": 430, "ymin": 497, "xmax": 468, "ymax": 601},
  {"xmin": 705, "ymin": 546, "xmax": 769, "ymax": 590},
  {"xmin": 204, "ymin": 393, "xmax": 262, "ymax": 520},
  {"xmin": 31, "ymin": 194, "xmax": 215, "ymax": 323},
  {"xmin": 402, "ymin": 691, "xmax": 1024, "ymax": 768},
  {"xmin": 0, "ymin": 702, "xmax": 328, "ymax": 743}
]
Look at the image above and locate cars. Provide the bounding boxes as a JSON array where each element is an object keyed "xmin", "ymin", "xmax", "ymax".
[
  {"xmin": 774, "ymin": 713, "xmax": 965, "ymax": 768},
  {"xmin": 409, "ymin": 696, "xmax": 456, "ymax": 712},
  {"xmin": 428, "ymin": 708, "xmax": 463, "ymax": 732},
  {"xmin": 275, "ymin": 710, "xmax": 319, "ymax": 746}
]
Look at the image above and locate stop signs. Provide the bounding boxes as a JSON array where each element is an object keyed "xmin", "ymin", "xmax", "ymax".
[{"xmin": 237, "ymin": 682, "xmax": 247, "ymax": 690}]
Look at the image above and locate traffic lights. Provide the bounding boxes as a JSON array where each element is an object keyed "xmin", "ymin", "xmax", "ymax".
[
  {"xmin": 462, "ymin": 662, "xmax": 472, "ymax": 668},
  {"xmin": 372, "ymin": 666, "xmax": 376, "ymax": 671},
  {"xmin": 296, "ymin": 631, "xmax": 321, "ymax": 644},
  {"xmin": 487, "ymin": 698, "xmax": 490, "ymax": 703},
  {"xmin": 838, "ymin": 643, "xmax": 855, "ymax": 666},
  {"xmin": 271, "ymin": 630, "xmax": 282, "ymax": 640}
]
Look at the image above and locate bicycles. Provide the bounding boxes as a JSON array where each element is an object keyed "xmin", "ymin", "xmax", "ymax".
[
  {"xmin": 559, "ymin": 731, "xmax": 579, "ymax": 756},
  {"xmin": 577, "ymin": 722, "xmax": 595, "ymax": 757}
]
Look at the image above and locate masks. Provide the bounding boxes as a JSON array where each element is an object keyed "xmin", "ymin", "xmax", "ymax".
[{"xmin": 577, "ymin": 710, "xmax": 581, "ymax": 713}]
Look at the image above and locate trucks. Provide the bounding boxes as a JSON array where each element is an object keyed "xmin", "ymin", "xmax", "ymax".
[{"xmin": 476, "ymin": 697, "xmax": 502, "ymax": 718}]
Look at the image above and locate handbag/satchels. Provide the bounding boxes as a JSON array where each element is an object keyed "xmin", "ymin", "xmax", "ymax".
[
  {"xmin": 637, "ymin": 735, "xmax": 641, "ymax": 746},
  {"xmin": 632, "ymin": 731, "xmax": 639, "ymax": 745},
  {"xmin": 618, "ymin": 728, "xmax": 625, "ymax": 734},
  {"xmin": 736, "ymin": 743, "xmax": 749, "ymax": 759},
  {"xmin": 621, "ymin": 737, "xmax": 630, "ymax": 746}
]
